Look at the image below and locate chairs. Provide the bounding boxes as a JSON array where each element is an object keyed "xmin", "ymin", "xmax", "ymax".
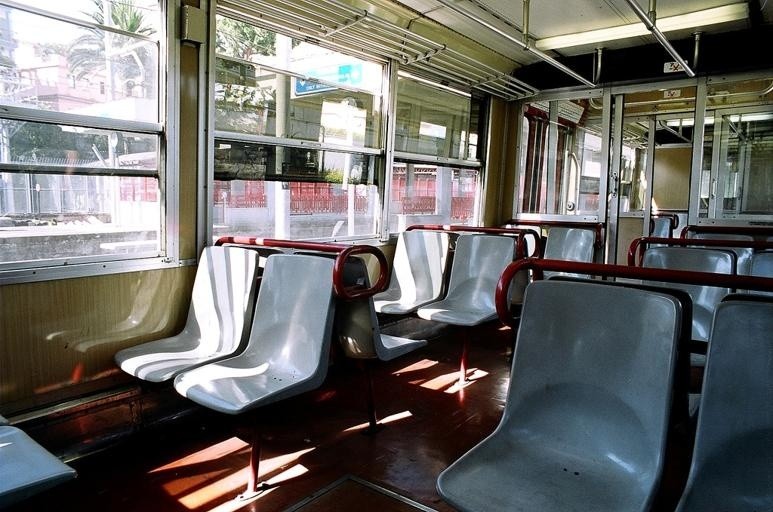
[
  {"xmin": 108, "ymin": 227, "xmax": 429, "ymax": 502},
  {"xmin": 438, "ymin": 256, "xmax": 773, "ymax": 512},
  {"xmin": 371, "ymin": 209, "xmax": 681, "ymax": 376},
  {"xmin": 625, "ymin": 234, "xmax": 772, "ymax": 358},
  {"xmin": 0, "ymin": 409, "xmax": 81, "ymax": 511},
  {"xmin": 679, "ymin": 224, "xmax": 772, "ymax": 298}
]
